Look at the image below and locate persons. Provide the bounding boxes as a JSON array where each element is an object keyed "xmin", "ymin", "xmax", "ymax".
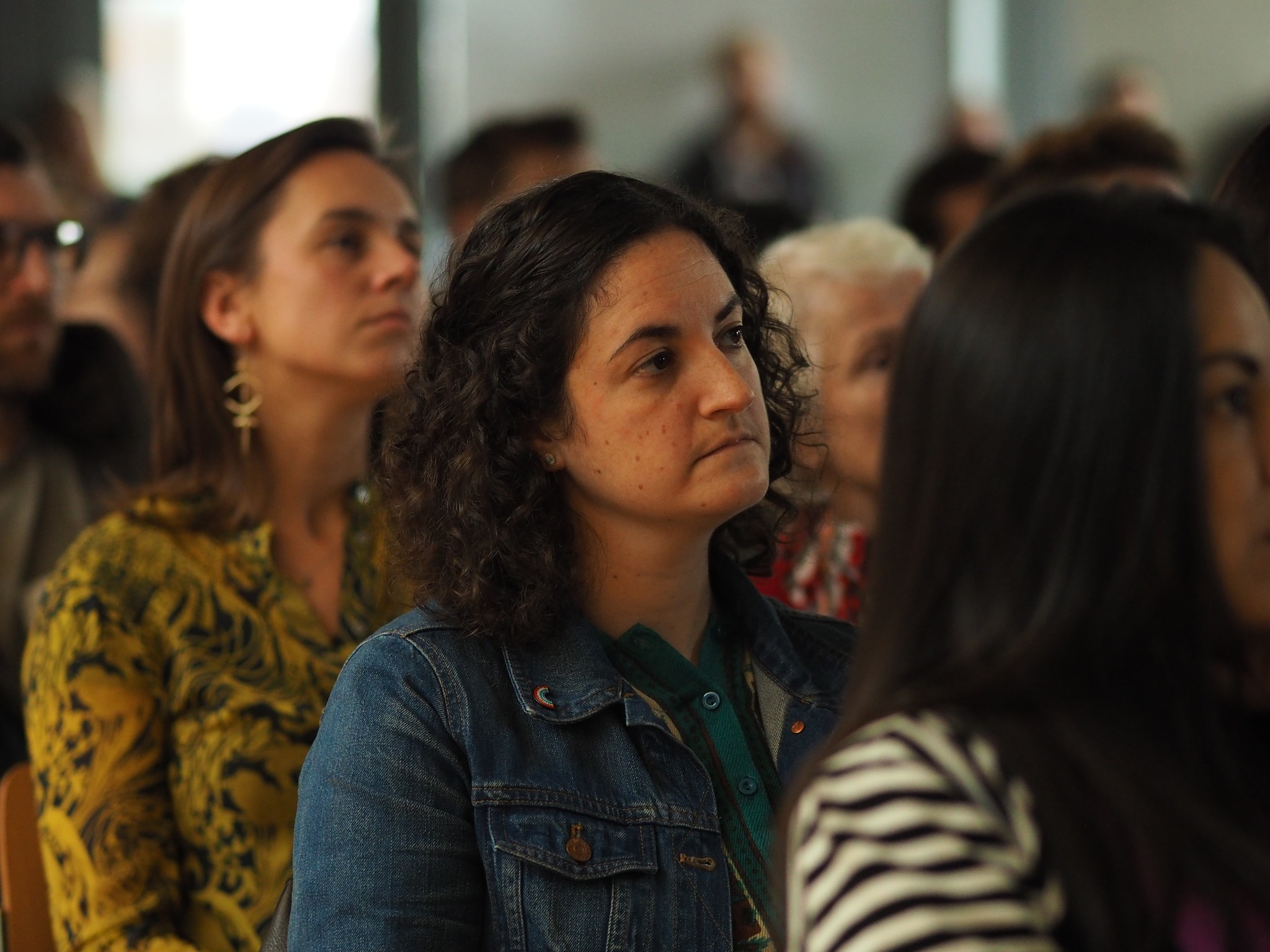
[
  {"xmin": 883, "ymin": 63, "xmax": 1195, "ymax": 258},
  {"xmin": 283, "ymin": 175, "xmax": 858, "ymax": 952},
  {"xmin": 665, "ymin": 48, "xmax": 842, "ymax": 246},
  {"xmin": 772, "ymin": 188, "xmax": 1270, "ymax": 952},
  {"xmin": 751, "ymin": 208, "xmax": 936, "ymax": 625},
  {"xmin": 433, "ymin": 109, "xmax": 594, "ymax": 242},
  {"xmin": 2, "ymin": 83, "xmax": 423, "ymax": 951}
]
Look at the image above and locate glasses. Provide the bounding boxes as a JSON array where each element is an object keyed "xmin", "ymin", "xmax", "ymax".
[{"xmin": 0, "ymin": 211, "xmax": 86, "ymax": 280}]
